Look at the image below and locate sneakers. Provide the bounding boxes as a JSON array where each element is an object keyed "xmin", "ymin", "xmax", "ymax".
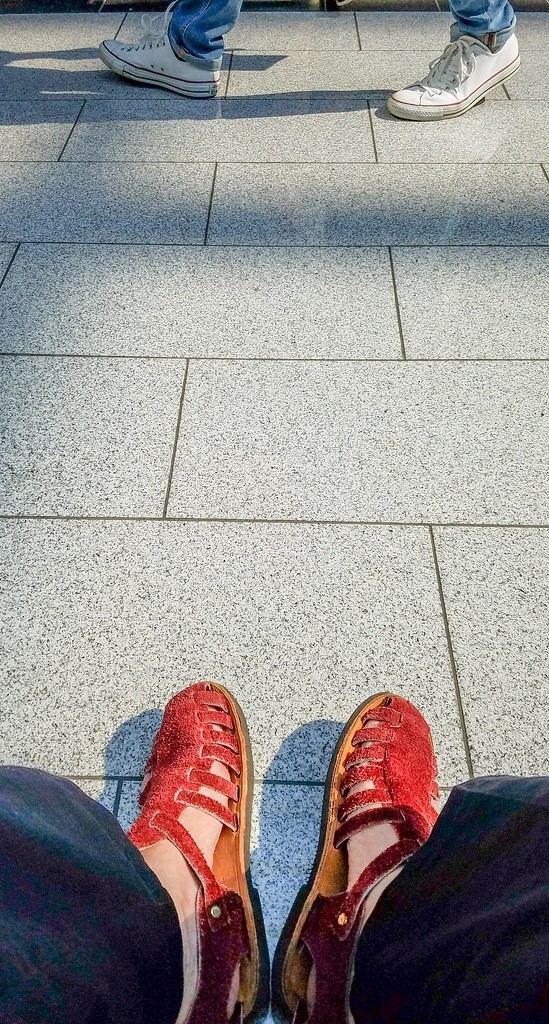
[
  {"xmin": 98, "ymin": 34, "xmax": 220, "ymax": 100},
  {"xmin": 387, "ymin": 34, "xmax": 521, "ymax": 120}
]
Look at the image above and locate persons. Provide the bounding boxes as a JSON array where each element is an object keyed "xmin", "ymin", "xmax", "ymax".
[
  {"xmin": 1, "ymin": 684, "xmax": 549, "ymax": 1023},
  {"xmin": 97, "ymin": 1, "xmax": 522, "ymax": 123}
]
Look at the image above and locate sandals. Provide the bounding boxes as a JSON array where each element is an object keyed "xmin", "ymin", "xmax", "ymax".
[
  {"xmin": 127, "ymin": 681, "xmax": 271, "ymax": 1024},
  {"xmin": 272, "ymin": 692, "xmax": 439, "ymax": 1024}
]
